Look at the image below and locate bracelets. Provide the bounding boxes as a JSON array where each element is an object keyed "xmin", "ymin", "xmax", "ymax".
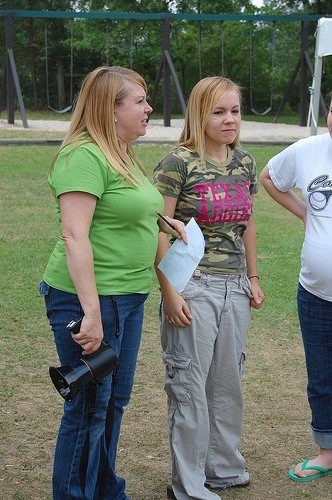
[{"xmin": 248, "ymin": 275, "xmax": 259, "ymax": 280}]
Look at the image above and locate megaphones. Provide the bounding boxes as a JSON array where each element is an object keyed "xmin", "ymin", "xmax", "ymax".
[{"xmin": 49, "ymin": 317, "xmax": 119, "ymax": 401}]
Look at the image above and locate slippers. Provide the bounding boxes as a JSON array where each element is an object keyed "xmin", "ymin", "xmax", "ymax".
[{"xmin": 288, "ymin": 460, "xmax": 332, "ymax": 483}]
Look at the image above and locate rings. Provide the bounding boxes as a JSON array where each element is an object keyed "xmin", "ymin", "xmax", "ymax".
[{"xmin": 168, "ymin": 320, "xmax": 175, "ymax": 324}]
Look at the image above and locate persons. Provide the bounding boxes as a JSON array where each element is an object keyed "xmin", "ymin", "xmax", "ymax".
[
  {"xmin": 259, "ymin": 90, "xmax": 332, "ymax": 482},
  {"xmin": 40, "ymin": 66, "xmax": 188, "ymax": 500},
  {"xmin": 152, "ymin": 76, "xmax": 265, "ymax": 500}
]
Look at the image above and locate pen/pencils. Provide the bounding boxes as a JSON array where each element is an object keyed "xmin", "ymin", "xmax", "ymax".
[{"xmin": 155, "ymin": 211, "xmax": 175, "ymax": 230}]
